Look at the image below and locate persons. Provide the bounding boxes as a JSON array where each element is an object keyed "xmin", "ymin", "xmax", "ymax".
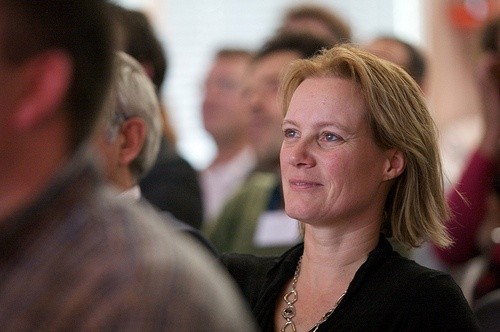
[
  {"xmin": 0, "ymin": 0, "xmax": 257, "ymax": 332},
  {"xmin": 431, "ymin": 17, "xmax": 500, "ymax": 304},
  {"xmin": 78, "ymin": 6, "xmax": 221, "ymax": 262},
  {"xmin": 199, "ymin": 3, "xmax": 425, "ymax": 260},
  {"xmin": 220, "ymin": 43, "xmax": 480, "ymax": 332}
]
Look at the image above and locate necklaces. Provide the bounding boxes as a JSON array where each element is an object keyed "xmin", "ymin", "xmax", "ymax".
[{"xmin": 281, "ymin": 256, "xmax": 348, "ymax": 332}]
